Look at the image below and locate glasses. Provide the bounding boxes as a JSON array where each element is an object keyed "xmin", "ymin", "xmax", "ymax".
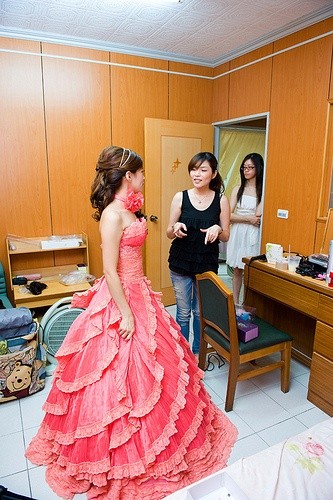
[{"xmin": 240, "ymin": 167, "xmax": 255, "ymax": 171}]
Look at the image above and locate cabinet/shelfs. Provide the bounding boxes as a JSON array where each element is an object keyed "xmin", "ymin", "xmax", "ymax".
[
  {"xmin": 5, "ymin": 230, "xmax": 91, "ymax": 312},
  {"xmin": 241, "ymin": 252, "xmax": 333, "ymax": 418}
]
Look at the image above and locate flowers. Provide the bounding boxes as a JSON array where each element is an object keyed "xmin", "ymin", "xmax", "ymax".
[{"xmin": 123, "ymin": 189, "xmax": 145, "ymax": 213}]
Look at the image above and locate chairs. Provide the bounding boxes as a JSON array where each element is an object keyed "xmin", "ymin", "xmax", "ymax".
[{"xmin": 195, "ymin": 271, "xmax": 293, "ymax": 413}]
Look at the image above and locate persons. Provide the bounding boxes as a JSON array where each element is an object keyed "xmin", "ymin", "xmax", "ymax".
[
  {"xmin": 24, "ymin": 145, "xmax": 239, "ymax": 500},
  {"xmin": 225, "ymin": 152, "xmax": 264, "ymax": 304},
  {"xmin": 166, "ymin": 152, "xmax": 231, "ymax": 360}
]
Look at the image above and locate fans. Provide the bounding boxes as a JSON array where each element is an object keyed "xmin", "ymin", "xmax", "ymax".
[{"xmin": 38, "ymin": 296, "xmax": 85, "ymax": 376}]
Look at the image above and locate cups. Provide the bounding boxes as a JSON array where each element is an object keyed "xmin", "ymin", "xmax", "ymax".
[{"xmin": 287, "ymin": 255, "xmax": 301, "ymax": 272}]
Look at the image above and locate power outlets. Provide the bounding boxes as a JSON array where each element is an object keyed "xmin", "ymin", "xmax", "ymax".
[{"xmin": 276, "ymin": 208, "xmax": 289, "ymax": 219}]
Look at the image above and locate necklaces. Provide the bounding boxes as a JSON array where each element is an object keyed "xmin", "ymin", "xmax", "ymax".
[{"xmin": 198, "ymin": 194, "xmax": 207, "ymax": 203}]
